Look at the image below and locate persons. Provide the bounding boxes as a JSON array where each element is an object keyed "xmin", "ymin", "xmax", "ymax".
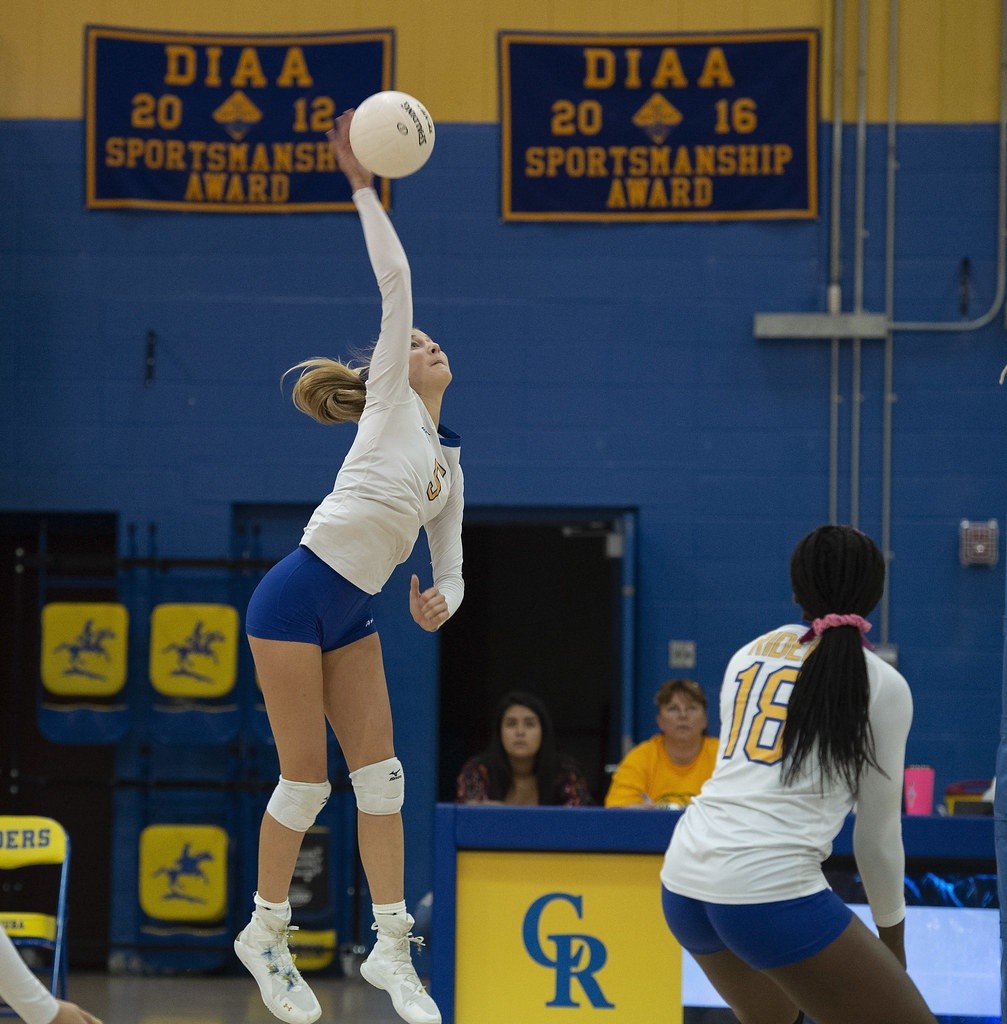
[
  {"xmin": 661, "ymin": 524, "xmax": 938, "ymax": 1024},
  {"xmin": 457, "ymin": 692, "xmax": 594, "ymax": 806},
  {"xmin": 233, "ymin": 108, "xmax": 465, "ymax": 1024},
  {"xmin": 0, "ymin": 921, "xmax": 101, "ymax": 1024},
  {"xmin": 605, "ymin": 679, "xmax": 719, "ymax": 807}
]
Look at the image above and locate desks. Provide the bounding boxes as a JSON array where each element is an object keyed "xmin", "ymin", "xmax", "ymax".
[{"xmin": 424, "ymin": 797, "xmax": 1007, "ymax": 1024}]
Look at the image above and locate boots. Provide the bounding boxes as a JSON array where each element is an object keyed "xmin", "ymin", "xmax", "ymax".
[
  {"xmin": 360, "ymin": 912, "xmax": 442, "ymax": 1024},
  {"xmin": 234, "ymin": 905, "xmax": 322, "ymax": 1024}
]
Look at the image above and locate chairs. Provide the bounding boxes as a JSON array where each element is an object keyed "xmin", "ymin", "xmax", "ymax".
[{"xmin": 0, "ymin": 814, "xmax": 69, "ymax": 1024}]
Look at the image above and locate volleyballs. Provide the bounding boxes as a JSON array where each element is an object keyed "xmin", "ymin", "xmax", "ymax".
[{"xmin": 349, "ymin": 89, "xmax": 437, "ymax": 179}]
